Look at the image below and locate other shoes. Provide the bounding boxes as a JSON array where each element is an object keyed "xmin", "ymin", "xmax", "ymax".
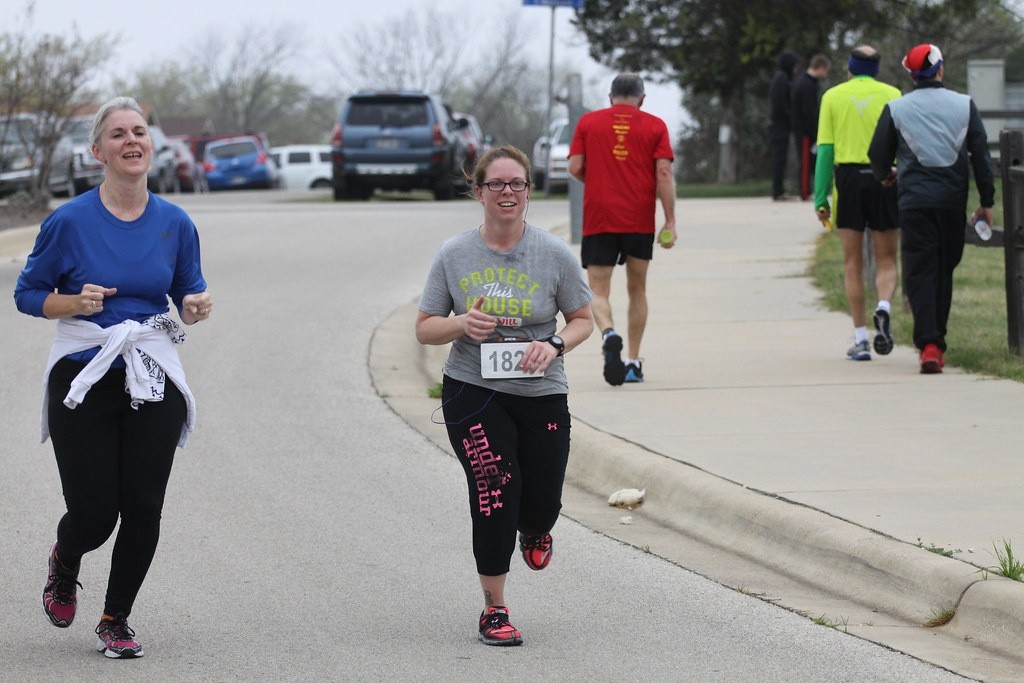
[{"xmin": 920, "ymin": 343, "xmax": 943, "ymax": 374}]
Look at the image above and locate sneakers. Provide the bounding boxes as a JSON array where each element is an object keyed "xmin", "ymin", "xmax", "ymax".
[
  {"xmin": 625, "ymin": 361, "xmax": 643, "ymax": 383},
  {"xmin": 603, "ymin": 331, "xmax": 626, "ymax": 385},
  {"xmin": 872, "ymin": 308, "xmax": 893, "ymax": 355},
  {"xmin": 95, "ymin": 618, "xmax": 143, "ymax": 658},
  {"xmin": 518, "ymin": 531, "xmax": 554, "ymax": 570},
  {"xmin": 847, "ymin": 338, "xmax": 872, "ymax": 359},
  {"xmin": 43, "ymin": 540, "xmax": 84, "ymax": 627},
  {"xmin": 479, "ymin": 604, "xmax": 522, "ymax": 645}
]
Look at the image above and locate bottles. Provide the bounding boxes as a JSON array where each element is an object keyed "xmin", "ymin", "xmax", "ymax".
[
  {"xmin": 659, "ymin": 230, "xmax": 675, "ymax": 246},
  {"xmin": 971, "ymin": 212, "xmax": 992, "ymax": 240}
]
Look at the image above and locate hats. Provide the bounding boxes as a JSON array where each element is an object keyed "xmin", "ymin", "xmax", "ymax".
[
  {"xmin": 848, "ymin": 44, "xmax": 880, "ymax": 77},
  {"xmin": 901, "ymin": 44, "xmax": 944, "ymax": 79}
]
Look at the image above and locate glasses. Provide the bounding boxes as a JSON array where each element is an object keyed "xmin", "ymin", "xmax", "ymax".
[{"xmin": 482, "ymin": 178, "xmax": 529, "ymax": 192}]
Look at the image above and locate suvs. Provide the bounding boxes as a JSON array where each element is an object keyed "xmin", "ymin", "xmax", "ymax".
[
  {"xmin": 63, "ymin": 113, "xmax": 195, "ymax": 192},
  {"xmin": 453, "ymin": 110, "xmax": 495, "ymax": 192},
  {"xmin": 331, "ymin": 88, "xmax": 456, "ymax": 201},
  {"xmin": 531, "ymin": 116, "xmax": 572, "ymax": 194}
]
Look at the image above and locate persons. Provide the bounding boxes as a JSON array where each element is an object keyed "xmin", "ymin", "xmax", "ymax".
[
  {"xmin": 790, "ymin": 53, "xmax": 834, "ymax": 200},
  {"xmin": 13, "ymin": 98, "xmax": 212, "ymax": 659},
  {"xmin": 769, "ymin": 53, "xmax": 799, "ymax": 201},
  {"xmin": 565, "ymin": 74, "xmax": 678, "ymax": 388},
  {"xmin": 813, "ymin": 45, "xmax": 903, "ymax": 361},
  {"xmin": 867, "ymin": 44, "xmax": 996, "ymax": 375},
  {"xmin": 415, "ymin": 142, "xmax": 597, "ymax": 645}
]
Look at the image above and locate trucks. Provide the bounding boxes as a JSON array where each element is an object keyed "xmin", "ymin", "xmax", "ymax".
[{"xmin": 169, "ymin": 132, "xmax": 269, "ymax": 177}]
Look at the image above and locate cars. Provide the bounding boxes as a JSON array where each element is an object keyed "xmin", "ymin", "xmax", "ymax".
[{"xmin": 201, "ymin": 135, "xmax": 277, "ymax": 192}]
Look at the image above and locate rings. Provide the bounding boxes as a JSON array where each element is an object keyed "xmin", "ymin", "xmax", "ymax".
[
  {"xmin": 205, "ymin": 307, "xmax": 208, "ymax": 314},
  {"xmin": 92, "ymin": 300, "xmax": 96, "ymax": 308}
]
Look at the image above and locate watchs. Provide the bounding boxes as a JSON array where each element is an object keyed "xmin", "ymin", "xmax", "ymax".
[{"xmin": 546, "ymin": 336, "xmax": 565, "ymax": 357}]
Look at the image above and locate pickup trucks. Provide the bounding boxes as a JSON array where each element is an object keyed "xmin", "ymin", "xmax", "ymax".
[{"xmin": 0, "ymin": 113, "xmax": 76, "ymax": 200}]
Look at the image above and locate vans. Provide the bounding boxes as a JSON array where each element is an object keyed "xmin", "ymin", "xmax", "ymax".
[{"xmin": 268, "ymin": 143, "xmax": 332, "ymax": 190}]
